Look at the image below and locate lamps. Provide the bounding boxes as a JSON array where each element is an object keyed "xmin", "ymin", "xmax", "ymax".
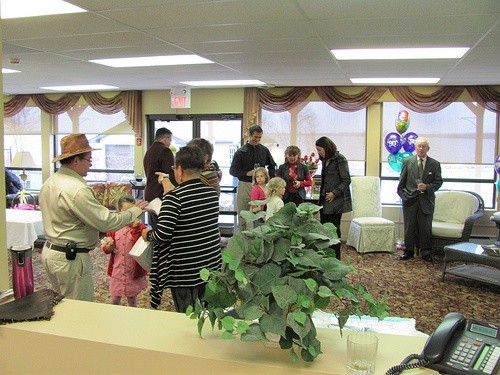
[{"xmin": 10, "ymin": 149, "xmax": 37, "ymax": 190}]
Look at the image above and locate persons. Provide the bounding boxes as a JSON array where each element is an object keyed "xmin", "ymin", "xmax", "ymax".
[
  {"xmin": 143, "ymin": 127, "xmax": 179, "ymax": 228},
  {"xmin": 155, "ymin": 138, "xmax": 220, "ymax": 197},
  {"xmin": 5, "ymin": 168, "xmax": 23, "ymax": 195},
  {"xmin": 141, "ymin": 145, "xmax": 223, "ymax": 314},
  {"xmin": 266, "ymin": 177, "xmax": 286, "ymax": 222},
  {"xmin": 229, "ymin": 124, "xmax": 276, "ymax": 230},
  {"xmin": 100, "ymin": 195, "xmax": 147, "ymax": 307},
  {"xmin": 38, "ymin": 134, "xmax": 148, "ymax": 302},
  {"xmin": 397, "ymin": 137, "xmax": 443, "ymax": 262},
  {"xmin": 315, "ymin": 136, "xmax": 352, "ymax": 259},
  {"xmin": 276, "ymin": 146, "xmax": 313, "ymax": 207},
  {"xmin": 249, "ymin": 167, "xmax": 269, "ymax": 215}
]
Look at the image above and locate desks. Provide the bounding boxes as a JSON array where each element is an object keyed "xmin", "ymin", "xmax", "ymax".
[
  {"xmin": 5, "ymin": 192, "xmax": 38, "ymax": 208},
  {"xmin": 4, "ymin": 207, "xmax": 44, "ymax": 252}
]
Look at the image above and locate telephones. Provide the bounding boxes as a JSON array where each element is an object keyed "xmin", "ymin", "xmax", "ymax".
[{"xmin": 420, "ymin": 310, "xmax": 500, "ymax": 375}]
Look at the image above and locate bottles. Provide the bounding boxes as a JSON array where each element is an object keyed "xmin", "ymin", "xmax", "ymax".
[{"xmin": 9, "ymin": 244, "xmax": 34, "ymax": 300}]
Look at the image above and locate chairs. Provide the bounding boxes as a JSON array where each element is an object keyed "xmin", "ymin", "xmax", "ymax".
[
  {"xmin": 11, "ymin": 189, "xmax": 36, "ymax": 210},
  {"xmin": 349, "ymin": 174, "xmax": 397, "ymax": 255}
]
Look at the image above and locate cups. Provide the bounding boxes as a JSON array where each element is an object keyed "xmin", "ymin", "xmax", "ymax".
[{"xmin": 346, "ymin": 332, "xmax": 379, "ymax": 375}]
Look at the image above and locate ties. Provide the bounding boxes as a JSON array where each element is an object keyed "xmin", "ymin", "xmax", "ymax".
[{"xmin": 418, "ymin": 158, "xmax": 424, "ymax": 177}]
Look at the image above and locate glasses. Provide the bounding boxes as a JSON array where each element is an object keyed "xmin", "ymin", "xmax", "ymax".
[{"xmin": 171, "ymin": 166, "xmax": 180, "ymax": 170}]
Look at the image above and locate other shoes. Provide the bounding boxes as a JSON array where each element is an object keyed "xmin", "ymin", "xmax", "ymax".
[
  {"xmin": 400, "ymin": 253, "xmax": 414, "ymax": 260},
  {"xmin": 422, "ymin": 255, "xmax": 432, "ymax": 262}
]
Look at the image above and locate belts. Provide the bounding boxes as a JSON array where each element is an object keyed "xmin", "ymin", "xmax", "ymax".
[{"xmin": 46, "ymin": 241, "xmax": 91, "ymax": 253}]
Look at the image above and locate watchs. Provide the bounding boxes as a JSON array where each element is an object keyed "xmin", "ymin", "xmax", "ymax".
[{"xmin": 160, "ymin": 176, "xmax": 169, "ymax": 183}]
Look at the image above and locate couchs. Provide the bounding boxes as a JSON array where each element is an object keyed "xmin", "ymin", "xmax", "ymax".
[
  {"xmin": 489, "ymin": 211, "xmax": 500, "ymax": 241},
  {"xmin": 416, "ymin": 186, "xmax": 486, "ymax": 252}
]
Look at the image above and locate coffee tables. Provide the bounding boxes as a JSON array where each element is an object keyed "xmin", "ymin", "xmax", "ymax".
[{"xmin": 439, "ymin": 241, "xmax": 500, "ymax": 291}]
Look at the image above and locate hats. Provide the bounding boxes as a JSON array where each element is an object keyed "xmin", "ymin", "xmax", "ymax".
[{"xmin": 51, "ymin": 133, "xmax": 102, "ymax": 162}]
